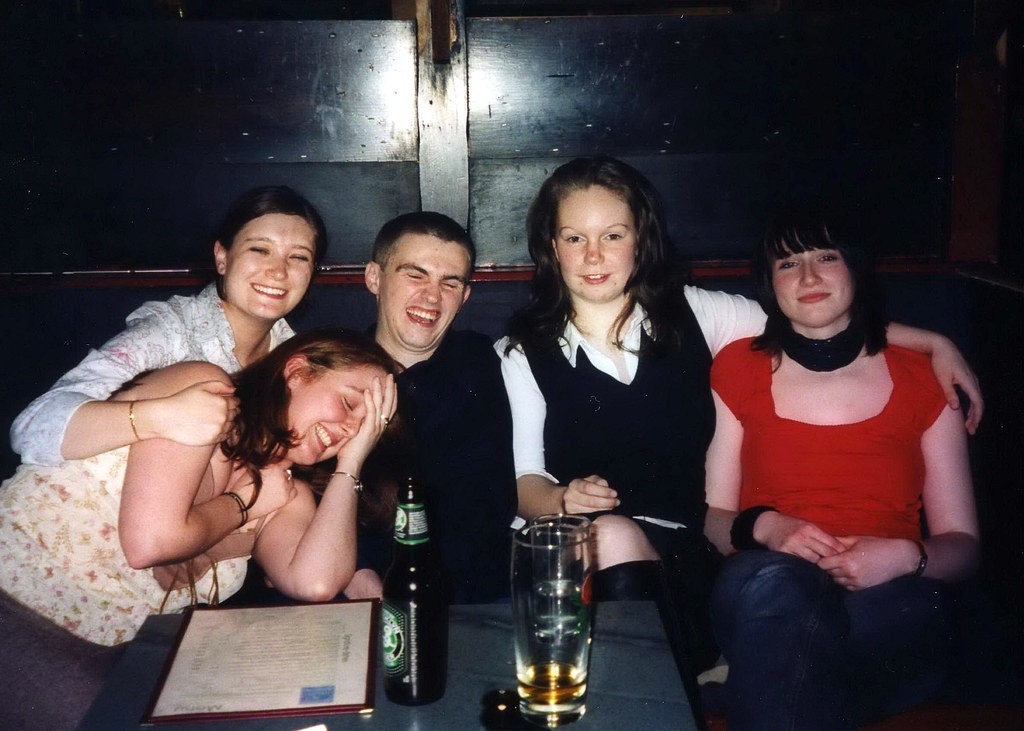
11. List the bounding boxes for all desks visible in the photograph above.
[75,591,699,731]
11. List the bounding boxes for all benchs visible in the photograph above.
[0,254,1023,731]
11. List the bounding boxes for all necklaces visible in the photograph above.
[778,321,865,371]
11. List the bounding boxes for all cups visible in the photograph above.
[510,524,591,727]
[519,515,592,652]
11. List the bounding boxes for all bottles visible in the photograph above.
[381,472,449,705]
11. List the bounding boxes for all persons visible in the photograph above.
[310,211,522,607]
[707,189,987,730]
[7,186,327,590]
[497,156,984,726]
[0,327,398,731]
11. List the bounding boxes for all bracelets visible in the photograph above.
[913,540,928,576]
[130,400,143,441]
[220,491,248,529]
[731,505,780,550]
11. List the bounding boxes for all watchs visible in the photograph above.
[328,472,363,500]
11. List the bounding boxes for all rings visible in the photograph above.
[381,415,389,425]
